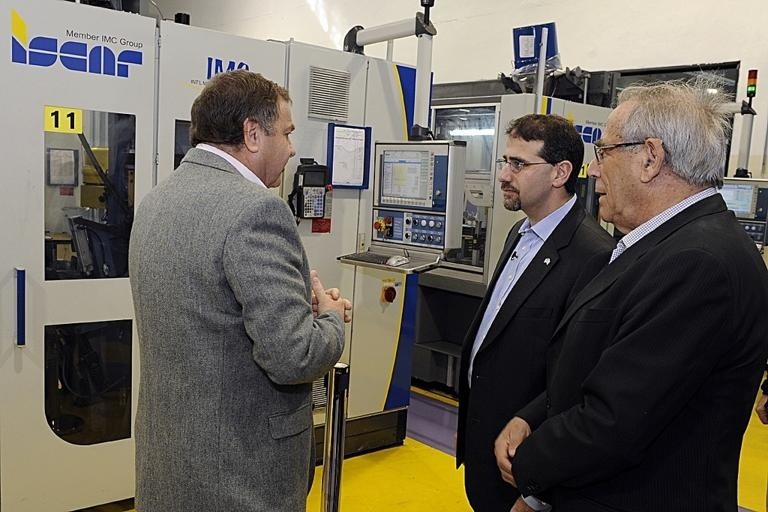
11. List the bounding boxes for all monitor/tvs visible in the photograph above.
[372,139,467,214]
[718,177,768,223]
[432,106,499,177]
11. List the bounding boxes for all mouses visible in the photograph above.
[386,254,409,268]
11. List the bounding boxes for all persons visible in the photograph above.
[490,81,768,512]
[453,111,621,511]
[126,69,355,510]
[755,373,768,425]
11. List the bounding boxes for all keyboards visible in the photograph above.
[336,251,390,266]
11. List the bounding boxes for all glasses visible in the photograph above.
[594,138,670,162]
[495,159,559,174]
